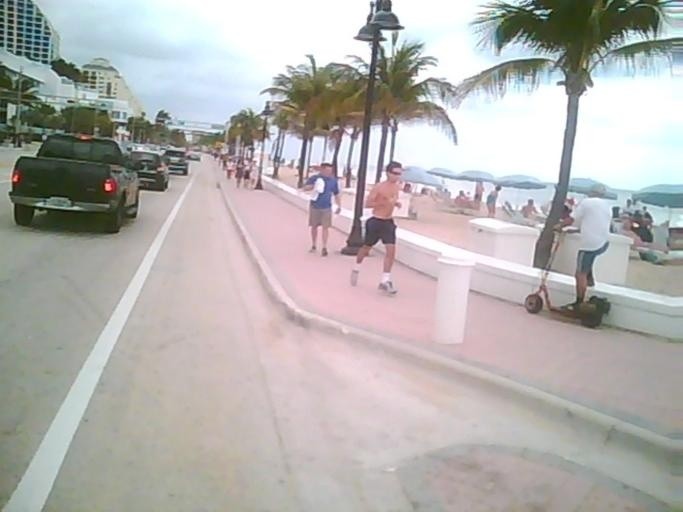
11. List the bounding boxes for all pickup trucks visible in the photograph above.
[8,132,140,235]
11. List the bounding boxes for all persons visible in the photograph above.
[220,153,258,188]
[552,183,612,303]
[403,181,654,246]
[303,163,342,256]
[350,160,402,294]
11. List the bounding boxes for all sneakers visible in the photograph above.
[587,279,596,286]
[322,248,328,255]
[562,304,582,308]
[308,246,320,253]
[378,281,398,294]
[351,269,358,287]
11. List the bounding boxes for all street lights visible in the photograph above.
[247,100,277,192]
[342,1,404,256]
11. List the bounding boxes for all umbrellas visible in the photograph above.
[632,184,683,208]
[554,178,617,200]
[426,168,546,189]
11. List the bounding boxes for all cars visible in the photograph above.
[116,141,205,191]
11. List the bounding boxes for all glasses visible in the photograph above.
[391,172,402,175]
